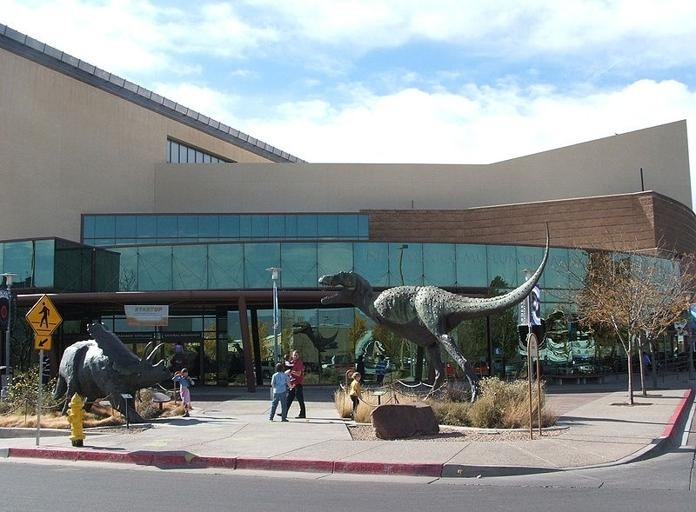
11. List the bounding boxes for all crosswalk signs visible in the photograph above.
[25,295,63,336]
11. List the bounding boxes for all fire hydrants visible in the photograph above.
[65,392,87,447]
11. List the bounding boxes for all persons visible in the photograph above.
[37,350,50,386]
[357,357,366,385]
[375,354,388,386]
[267,362,293,422]
[283,353,296,381]
[643,351,653,376]
[172,367,195,417]
[173,339,185,364]
[277,349,306,419]
[349,372,363,420]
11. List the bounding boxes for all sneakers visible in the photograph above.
[183,413,189,417]
[281,418,288,422]
[277,413,282,415]
[295,415,305,418]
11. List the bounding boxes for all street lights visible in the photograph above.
[265,267,282,364]
[0,273,17,374]
